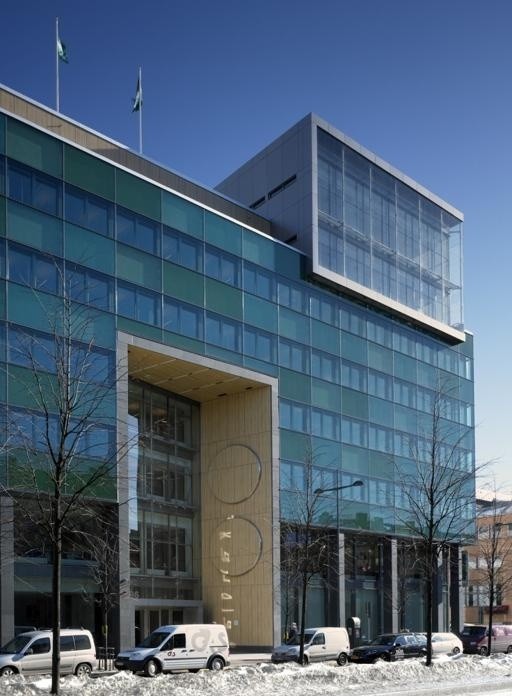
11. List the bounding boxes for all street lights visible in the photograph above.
[495,522,512,526]
[313,470,363,627]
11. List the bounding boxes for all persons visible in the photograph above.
[288,621,297,641]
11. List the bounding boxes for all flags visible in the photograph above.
[131,80,142,113]
[57,36,69,63]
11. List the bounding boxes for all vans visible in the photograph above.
[0,627,97,678]
[115,624,231,677]
[271,627,350,666]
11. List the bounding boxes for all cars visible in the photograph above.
[348,623,512,664]
